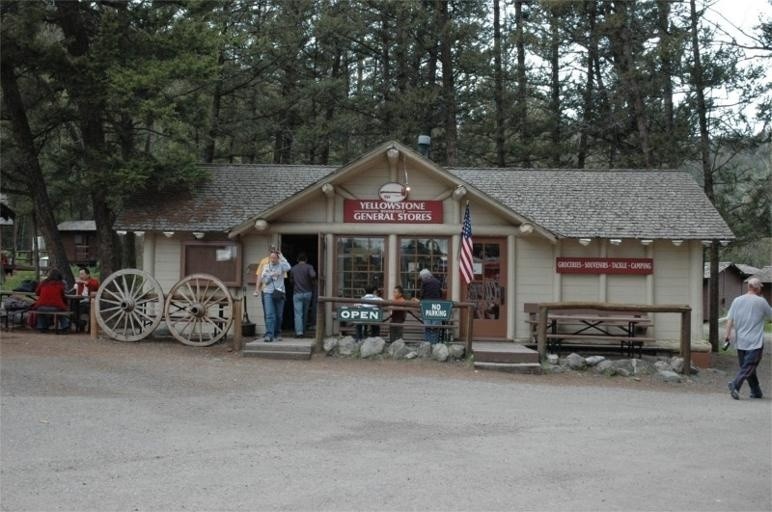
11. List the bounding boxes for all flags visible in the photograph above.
[458,202,474,285]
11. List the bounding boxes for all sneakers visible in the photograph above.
[727,381,740,400]
[40,320,87,333]
[263,334,306,341]
[750,393,763,399]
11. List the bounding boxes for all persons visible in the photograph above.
[260,250,292,341]
[32,267,72,334]
[724,278,772,401]
[0,252,10,276]
[289,253,317,338]
[410,268,444,344]
[252,244,288,339]
[64,267,100,334]
[389,284,407,344]
[356,283,381,337]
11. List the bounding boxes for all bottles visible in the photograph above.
[74,283,78,295]
[721,341,731,352]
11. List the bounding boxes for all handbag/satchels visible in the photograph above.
[272,289,285,301]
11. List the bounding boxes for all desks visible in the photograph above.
[546,314,650,356]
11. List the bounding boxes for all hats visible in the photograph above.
[747,278,764,288]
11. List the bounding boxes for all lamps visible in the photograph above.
[578,237,730,249]
[116,230,206,242]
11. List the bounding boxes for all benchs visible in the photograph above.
[524,320,654,358]
[537,333,655,358]
[4,290,96,333]
[349,322,457,342]
